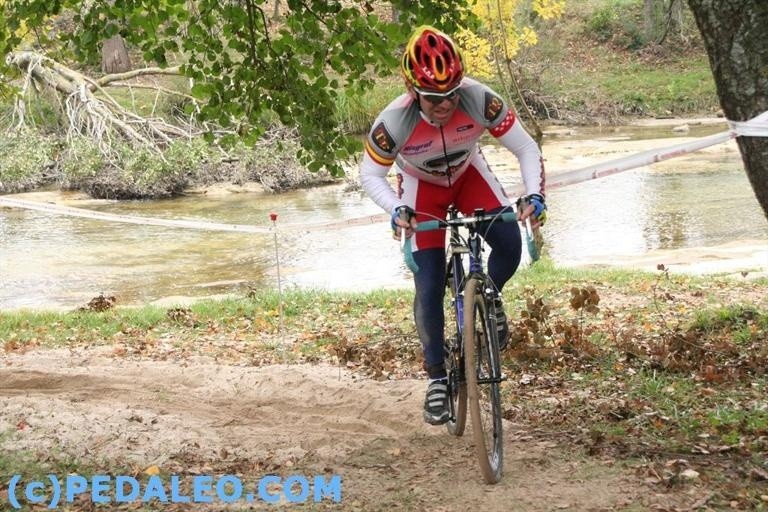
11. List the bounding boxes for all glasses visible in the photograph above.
[412,84,463,105]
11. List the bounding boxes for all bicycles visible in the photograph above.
[400,195,541,484]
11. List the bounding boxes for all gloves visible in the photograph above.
[390,204,416,242]
[526,194,549,227]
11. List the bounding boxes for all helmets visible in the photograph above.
[402,24,465,92]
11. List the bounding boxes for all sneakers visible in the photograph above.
[488,293,509,351]
[421,378,452,426]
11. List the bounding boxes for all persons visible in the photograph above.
[359,24,547,426]
